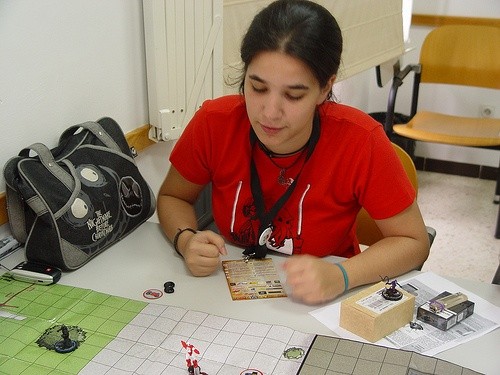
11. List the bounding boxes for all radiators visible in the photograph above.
[141,0,220,141]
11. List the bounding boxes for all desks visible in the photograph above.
[0,221,500,375]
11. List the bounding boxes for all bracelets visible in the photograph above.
[335,263,348,290]
[173,228,196,257]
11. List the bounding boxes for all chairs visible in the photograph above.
[355,143,436,271]
[386,24,500,238]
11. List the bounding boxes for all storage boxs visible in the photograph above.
[0,234,22,259]
[417,291,474,331]
[340,282,415,343]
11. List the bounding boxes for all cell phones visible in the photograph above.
[10,260,62,285]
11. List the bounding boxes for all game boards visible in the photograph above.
[0,272,489,375]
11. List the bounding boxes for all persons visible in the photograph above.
[155,0,429,306]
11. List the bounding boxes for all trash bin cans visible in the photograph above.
[367,112,411,152]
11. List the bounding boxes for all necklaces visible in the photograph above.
[261,145,306,184]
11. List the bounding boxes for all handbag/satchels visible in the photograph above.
[2,117,158,273]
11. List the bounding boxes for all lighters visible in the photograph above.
[429,292,468,312]
